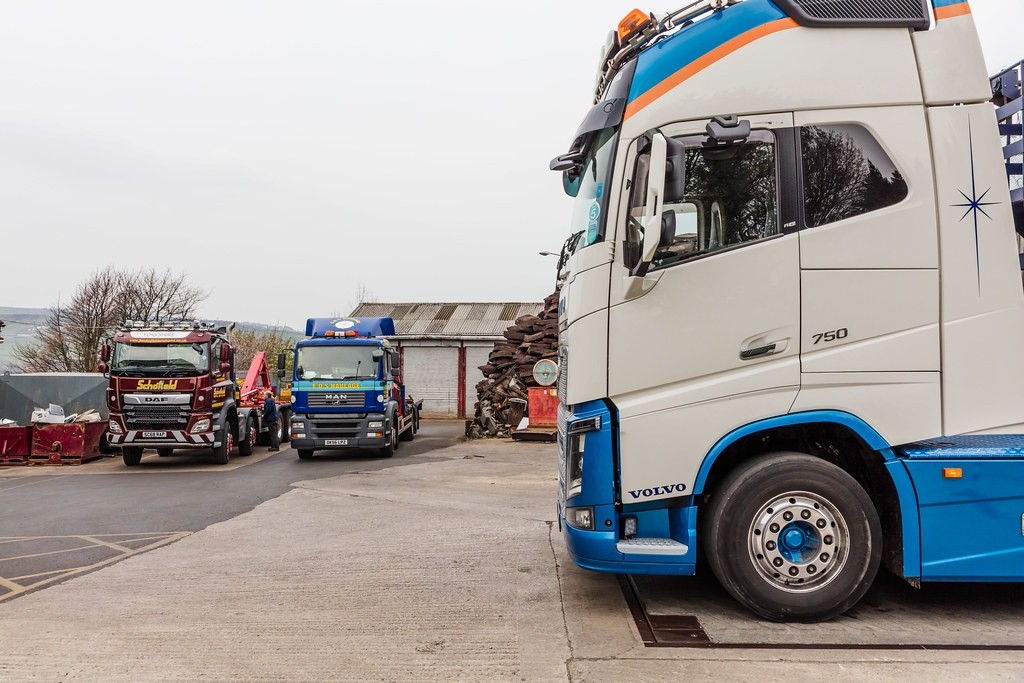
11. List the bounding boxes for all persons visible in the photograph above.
[262,392,279,451]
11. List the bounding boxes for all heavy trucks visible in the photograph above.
[554,0,1024,624]
[277,317,424,459]
[98,321,293,465]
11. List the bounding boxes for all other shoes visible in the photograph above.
[268,446,279,451]
